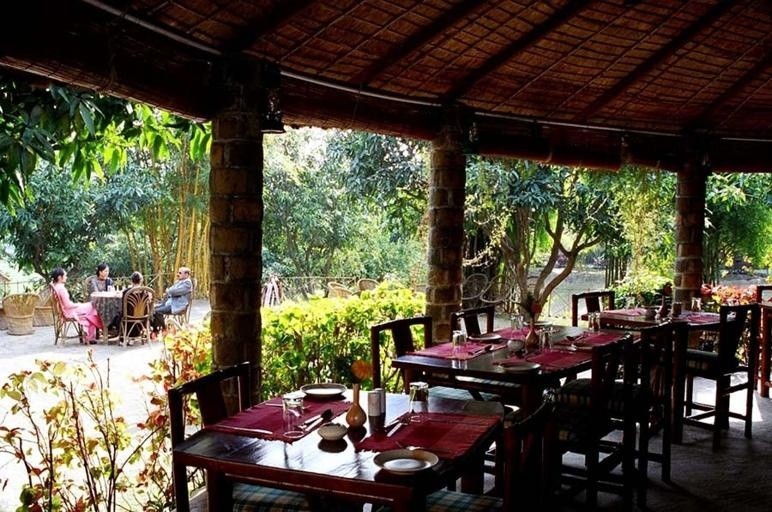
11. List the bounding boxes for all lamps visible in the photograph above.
[236,57,290,137]
[455,96,481,147]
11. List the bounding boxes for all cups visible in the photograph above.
[450,329,470,359]
[510,314,524,334]
[624,296,635,315]
[409,382,429,415]
[689,298,701,318]
[283,400,306,440]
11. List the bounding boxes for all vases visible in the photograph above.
[659,297,671,317]
[645,309,657,319]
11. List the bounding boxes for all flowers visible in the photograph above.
[518,294,548,317]
[334,355,373,384]
[641,290,659,309]
[659,283,674,296]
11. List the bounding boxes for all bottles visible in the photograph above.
[672,301,684,315]
[539,327,554,346]
[368,388,385,418]
[588,312,601,332]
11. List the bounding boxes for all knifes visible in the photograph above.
[216,423,273,435]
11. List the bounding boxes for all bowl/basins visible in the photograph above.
[316,423,348,439]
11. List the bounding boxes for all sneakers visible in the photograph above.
[84,329,167,347]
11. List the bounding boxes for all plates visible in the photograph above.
[570,342,603,350]
[470,333,503,342]
[371,445,440,475]
[523,320,552,327]
[300,380,348,397]
[499,359,542,373]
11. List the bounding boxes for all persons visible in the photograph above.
[50,267,104,345]
[117,270,149,347]
[87,263,114,302]
[148,267,193,335]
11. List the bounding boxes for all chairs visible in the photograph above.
[168,360,578,512]
[673,304,760,440]
[371,305,678,507]
[0,275,160,346]
[753,285,772,391]
[572,290,616,328]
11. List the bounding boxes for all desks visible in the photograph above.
[761,305,772,397]
[581,306,752,415]
